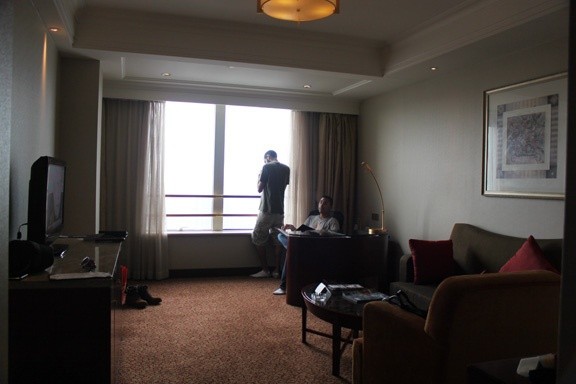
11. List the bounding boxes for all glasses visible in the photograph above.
[81,255,90,272]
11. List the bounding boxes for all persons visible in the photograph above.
[273,196,340,296]
[249,150,290,279]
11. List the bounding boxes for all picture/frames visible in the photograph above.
[481,71,568,200]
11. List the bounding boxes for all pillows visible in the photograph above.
[499,235,557,273]
[409,238,453,285]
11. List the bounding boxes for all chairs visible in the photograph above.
[308,211,344,233]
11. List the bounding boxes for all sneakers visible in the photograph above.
[271,272,279,278]
[248,270,269,278]
[272,288,285,294]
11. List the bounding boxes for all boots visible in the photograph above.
[124,286,147,308]
[138,285,161,304]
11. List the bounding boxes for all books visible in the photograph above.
[328,283,363,291]
[341,288,388,305]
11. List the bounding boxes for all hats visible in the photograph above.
[9,239,53,276]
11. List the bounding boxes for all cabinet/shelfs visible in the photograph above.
[9,235,122,384]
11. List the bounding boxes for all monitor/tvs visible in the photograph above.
[27,156,69,257]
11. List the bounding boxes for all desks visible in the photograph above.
[271,226,352,304]
[467,350,557,383]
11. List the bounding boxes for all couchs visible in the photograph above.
[389,223,564,317]
[350,270,562,383]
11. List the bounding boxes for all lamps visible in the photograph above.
[360,160,388,236]
[256,0,340,21]
